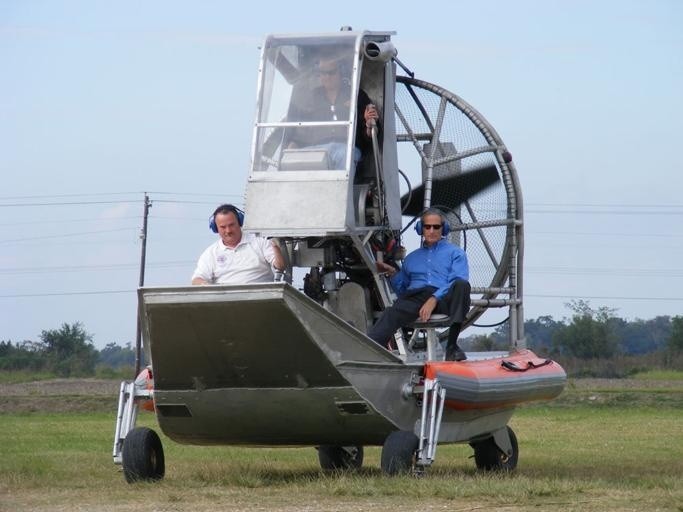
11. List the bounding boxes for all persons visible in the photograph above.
[192,204,290,285]
[366,207,471,361]
[287,54,379,182]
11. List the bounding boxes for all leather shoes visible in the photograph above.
[445,343,467,361]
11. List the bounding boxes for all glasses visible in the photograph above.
[424,224,443,230]
[330,104,338,121]
[320,69,337,76]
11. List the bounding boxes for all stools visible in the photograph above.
[407,313,449,365]
